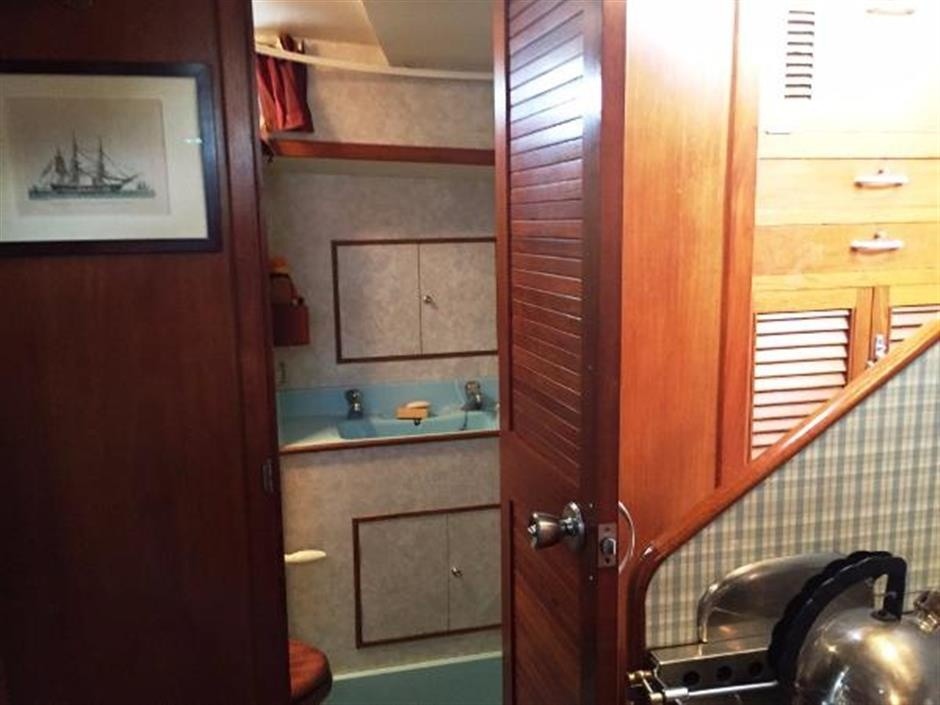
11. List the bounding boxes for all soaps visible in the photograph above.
[408,399,433,411]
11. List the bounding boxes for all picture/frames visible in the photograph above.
[1,56,225,260]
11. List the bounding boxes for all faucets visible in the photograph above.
[461,380,488,411]
[344,389,366,419]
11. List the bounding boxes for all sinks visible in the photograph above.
[337,409,504,444]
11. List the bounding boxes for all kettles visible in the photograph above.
[769,550,940,704]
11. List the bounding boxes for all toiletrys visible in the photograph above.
[269,255,298,309]
